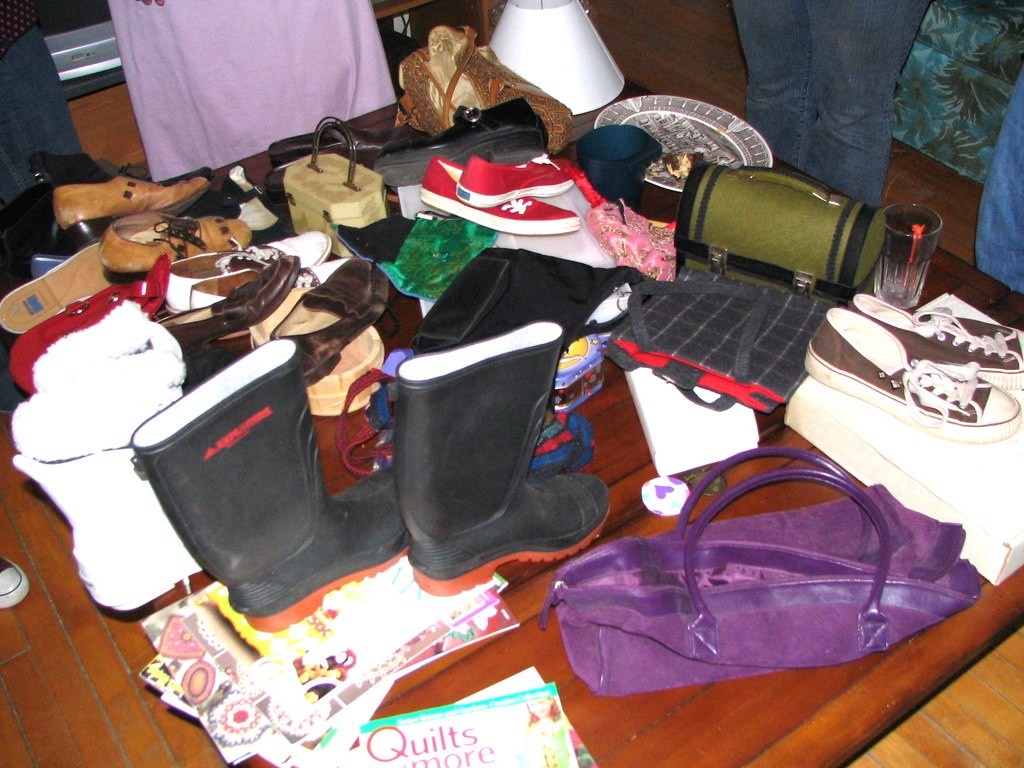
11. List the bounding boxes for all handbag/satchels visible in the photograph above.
[603,265,838,415]
[284,115,387,258]
[539,445,984,698]
[674,162,885,305]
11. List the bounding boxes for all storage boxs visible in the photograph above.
[555,334,606,413]
[784,292,1023,587]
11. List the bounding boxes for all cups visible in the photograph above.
[874,203,944,313]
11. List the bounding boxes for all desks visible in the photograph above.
[152,78,1024,768]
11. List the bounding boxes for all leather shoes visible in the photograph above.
[0,172,390,389]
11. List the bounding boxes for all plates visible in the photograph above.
[592,93,774,198]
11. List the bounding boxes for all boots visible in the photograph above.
[393,319,611,596]
[12,298,214,618]
[132,338,415,632]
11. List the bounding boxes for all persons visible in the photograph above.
[730,0,931,209]
[0,0,398,206]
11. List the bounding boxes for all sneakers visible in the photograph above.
[849,294,1024,391]
[803,308,1022,445]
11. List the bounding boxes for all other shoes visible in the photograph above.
[0,556,29,610]
[420,157,581,237]
[221,164,282,246]
[455,155,576,210]
[373,95,549,190]
[401,23,573,154]
